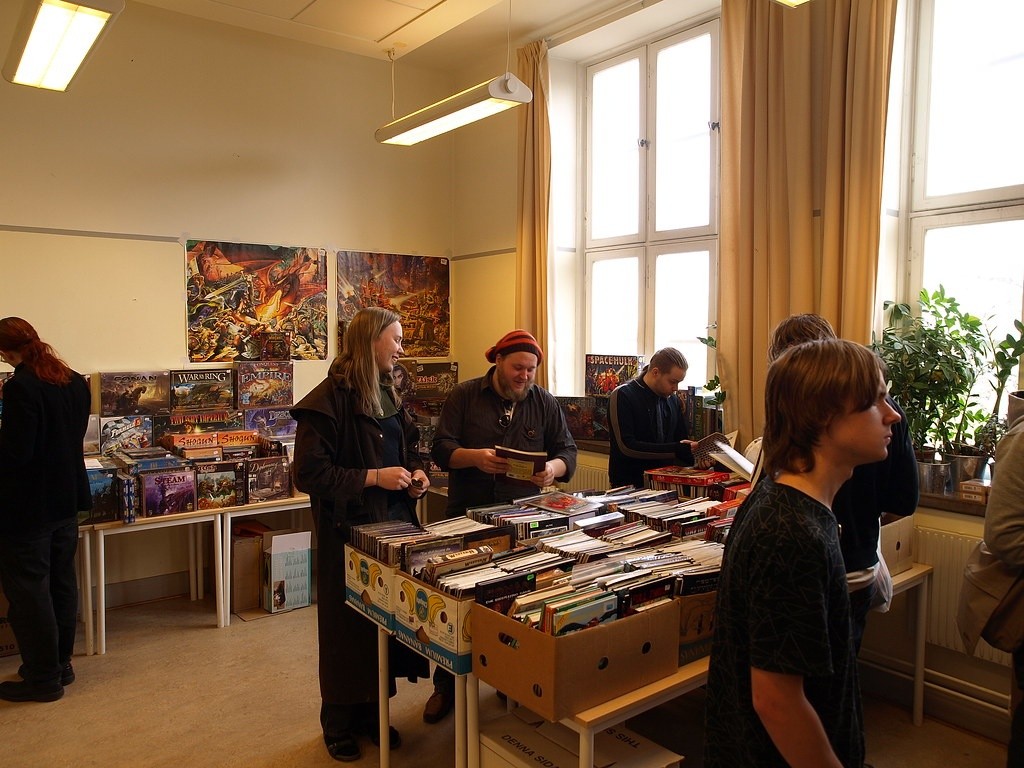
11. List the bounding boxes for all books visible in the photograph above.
[708,436,763,481]
[494,444,547,503]
[350,465,754,636]
[673,386,721,440]
[689,429,738,465]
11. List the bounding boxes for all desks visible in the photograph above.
[77,491,312,657]
[466,562,934,768]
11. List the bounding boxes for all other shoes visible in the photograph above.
[423,691,454,724]
[495,690,519,708]
[19,659,74,686]
[351,714,401,748]
[0,673,64,702]
[321,719,361,762]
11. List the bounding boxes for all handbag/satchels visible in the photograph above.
[956,541,1024,658]
[870,516,893,613]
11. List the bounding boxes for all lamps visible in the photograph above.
[0,0,126,93]
[374,0,533,147]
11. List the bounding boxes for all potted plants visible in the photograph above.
[866,284,1024,496]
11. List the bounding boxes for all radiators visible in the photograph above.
[551,464,610,494]
[904,526,1012,668]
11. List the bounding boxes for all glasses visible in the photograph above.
[410,478,424,491]
[498,399,514,428]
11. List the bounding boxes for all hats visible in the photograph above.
[485,329,543,366]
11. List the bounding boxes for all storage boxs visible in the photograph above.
[480,705,685,768]
[550,354,723,442]
[262,529,312,614]
[205,521,263,613]
[960,491,988,506]
[75,331,309,527]
[959,478,992,495]
[343,542,715,724]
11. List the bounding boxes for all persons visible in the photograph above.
[0,315,92,701]
[425,329,575,726]
[700,339,901,768]
[289,307,431,762]
[607,348,714,491]
[983,390,1024,768]
[750,314,919,655]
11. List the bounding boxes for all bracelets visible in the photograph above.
[375,466,380,487]
